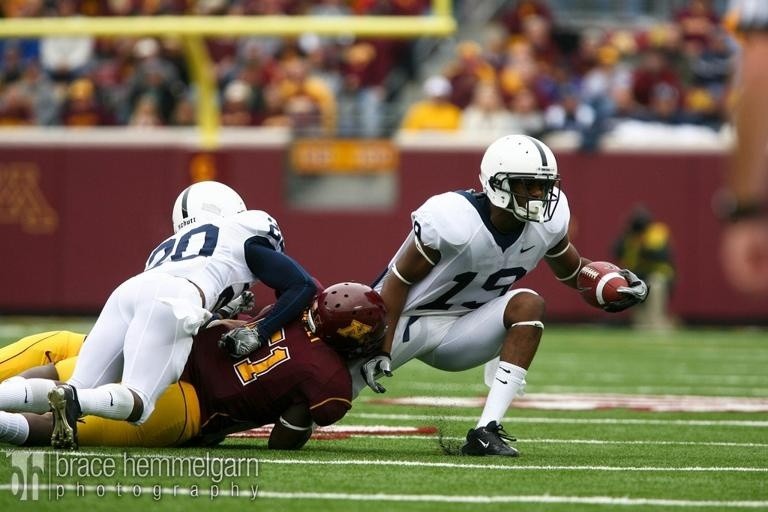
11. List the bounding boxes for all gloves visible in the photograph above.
[216,290,255,318]
[360,351,393,393]
[603,268,649,314]
[217,325,262,357]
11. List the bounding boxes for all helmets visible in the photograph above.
[478,133,556,223]
[171,180,248,234]
[306,281,389,359]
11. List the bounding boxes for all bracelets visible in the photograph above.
[719,192,768,226]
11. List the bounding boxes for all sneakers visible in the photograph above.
[47,384,83,451]
[462,420,520,456]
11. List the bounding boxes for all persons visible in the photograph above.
[0,179,318,429]
[713,0,768,297]
[0,309,256,383]
[0,281,392,450]
[342,132,649,458]
[617,209,681,331]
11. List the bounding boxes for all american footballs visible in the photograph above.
[577,262,629,309]
